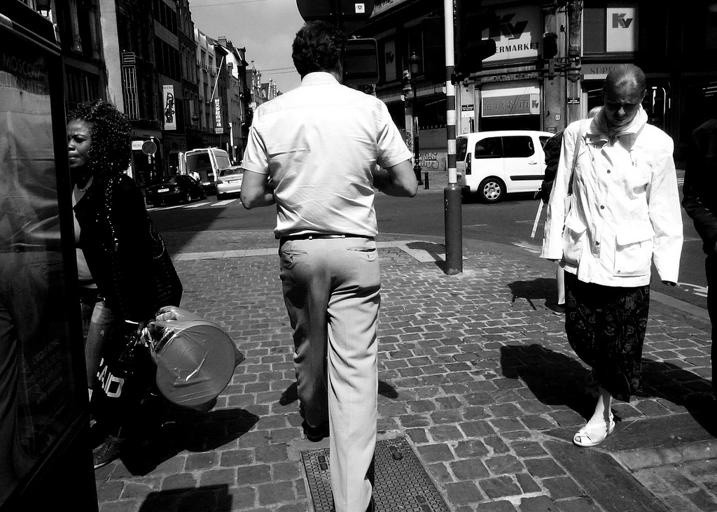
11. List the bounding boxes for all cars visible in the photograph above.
[215,166,246,202]
[144,174,208,205]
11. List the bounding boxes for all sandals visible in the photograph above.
[573,413,615,448]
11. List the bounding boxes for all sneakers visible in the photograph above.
[93,435,125,469]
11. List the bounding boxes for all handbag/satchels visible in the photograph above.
[540,129,574,204]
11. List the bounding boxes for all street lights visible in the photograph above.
[406,51,425,187]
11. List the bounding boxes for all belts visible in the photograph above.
[289,233,355,240]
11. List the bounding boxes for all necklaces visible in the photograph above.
[74,181,90,193]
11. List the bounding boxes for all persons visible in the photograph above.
[538,104,605,311]
[0,32,70,510]
[241,22,420,511]
[685,119,716,405]
[540,64,683,446]
[66,98,183,470]
[189,168,200,183]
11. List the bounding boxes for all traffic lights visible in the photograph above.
[545,33,558,58]
[457,0,496,72]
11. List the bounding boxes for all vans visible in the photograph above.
[456,126,559,207]
[177,147,232,187]
